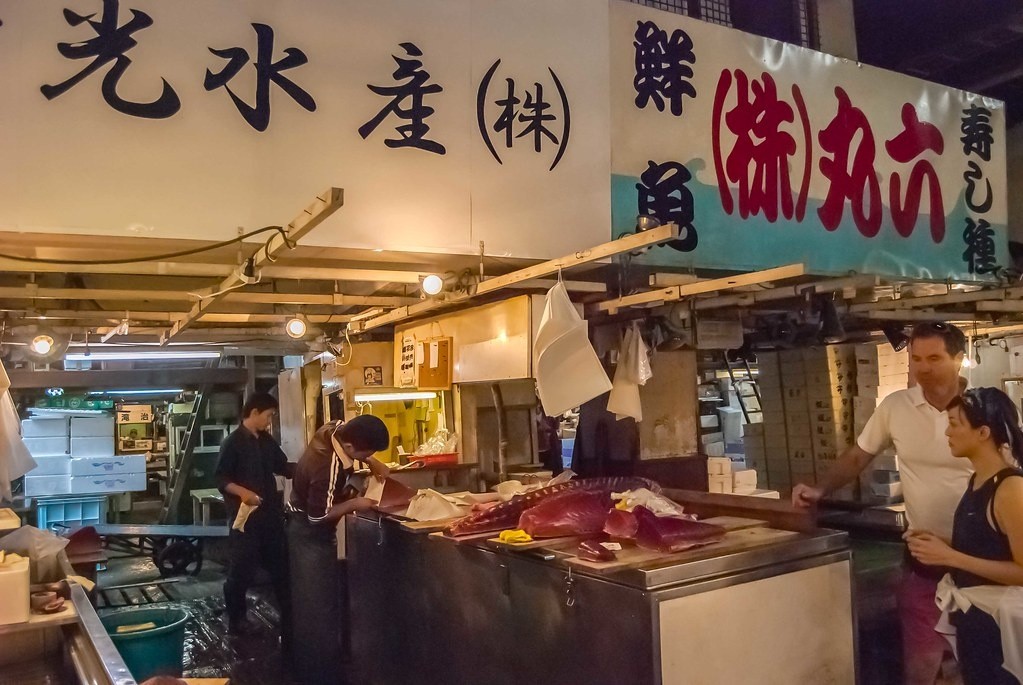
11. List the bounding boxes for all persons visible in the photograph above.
[902,387,1023,685]
[284,414,391,685]
[214,391,299,636]
[792,320,1023,685]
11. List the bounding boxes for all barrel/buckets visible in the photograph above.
[99,607,190,685]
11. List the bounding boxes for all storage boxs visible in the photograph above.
[20,408,147,571]
[697,346,910,507]
[1,557,30,625]
[30,553,57,583]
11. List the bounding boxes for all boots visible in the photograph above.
[224,582,264,635]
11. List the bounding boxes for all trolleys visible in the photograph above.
[50,522,202,578]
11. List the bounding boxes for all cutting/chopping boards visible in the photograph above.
[400,515,799,574]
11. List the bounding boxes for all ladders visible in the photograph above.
[725,354,761,424]
[160,359,218,526]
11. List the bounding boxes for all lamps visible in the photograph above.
[636,215,661,233]
[815,299,848,344]
[349,392,439,402]
[994,265,1023,283]
[880,325,909,352]
[32,326,57,354]
[287,319,322,339]
[63,350,221,361]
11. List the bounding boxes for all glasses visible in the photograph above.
[916,321,960,350]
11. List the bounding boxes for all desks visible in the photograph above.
[190,489,224,527]
